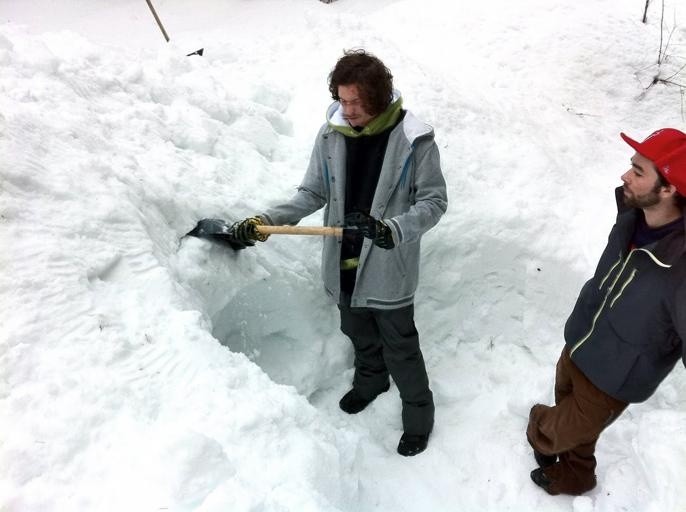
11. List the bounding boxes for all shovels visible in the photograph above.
[188,217,368,250]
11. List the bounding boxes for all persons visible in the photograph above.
[227,48,448,456]
[526,128,686,495]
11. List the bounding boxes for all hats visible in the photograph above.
[619,128,686,198]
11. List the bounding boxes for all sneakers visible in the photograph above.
[529,449,559,492]
[397,430,428,457]
[339,380,390,414]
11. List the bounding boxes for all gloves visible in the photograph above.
[226,214,270,248]
[348,211,395,250]
